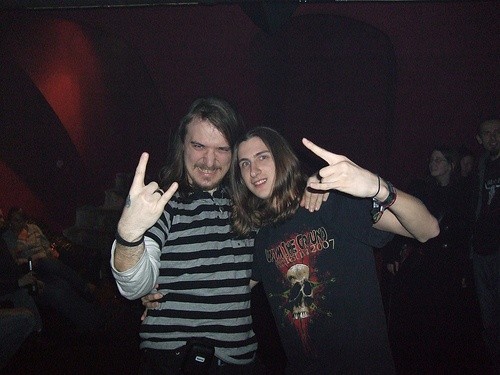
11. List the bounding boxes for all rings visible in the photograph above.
[154,188,163,197]
[314,168,324,181]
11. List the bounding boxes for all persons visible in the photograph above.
[141,126,442,375]
[386,116,500,375]
[0,206,108,340]
[109,98,330,375]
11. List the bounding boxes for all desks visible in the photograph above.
[62,227,123,306]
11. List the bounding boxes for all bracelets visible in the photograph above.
[113,230,144,246]
[371,172,398,224]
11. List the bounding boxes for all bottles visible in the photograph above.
[27,256,37,296]
